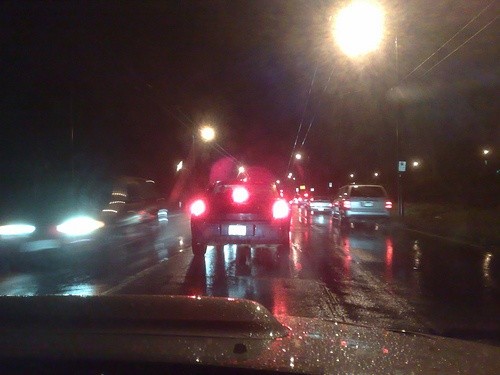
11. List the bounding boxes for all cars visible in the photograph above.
[289,193,315,208]
[307,195,332,215]
[0,176,169,274]
[190,180,292,256]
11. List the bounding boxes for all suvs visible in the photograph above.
[331,184,393,228]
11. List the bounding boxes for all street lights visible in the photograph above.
[335,3,404,219]
